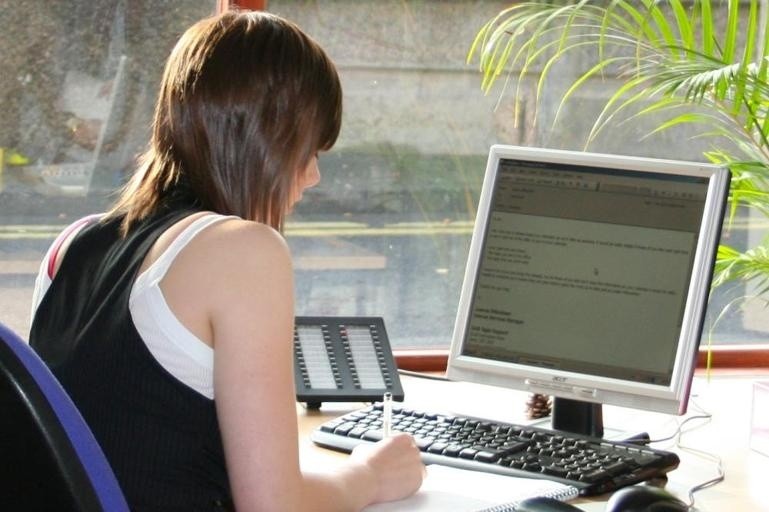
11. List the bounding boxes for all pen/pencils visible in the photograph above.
[383,392,393,440]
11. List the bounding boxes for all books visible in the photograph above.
[356,460,580,512]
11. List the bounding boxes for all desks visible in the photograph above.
[295,368,769,512]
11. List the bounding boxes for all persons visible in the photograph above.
[28,7,425,512]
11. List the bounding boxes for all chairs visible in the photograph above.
[0,316,132,512]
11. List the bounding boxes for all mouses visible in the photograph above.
[608,482,691,512]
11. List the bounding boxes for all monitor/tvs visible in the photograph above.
[445,144,733,444]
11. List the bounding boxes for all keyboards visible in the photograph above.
[312,401,679,495]
